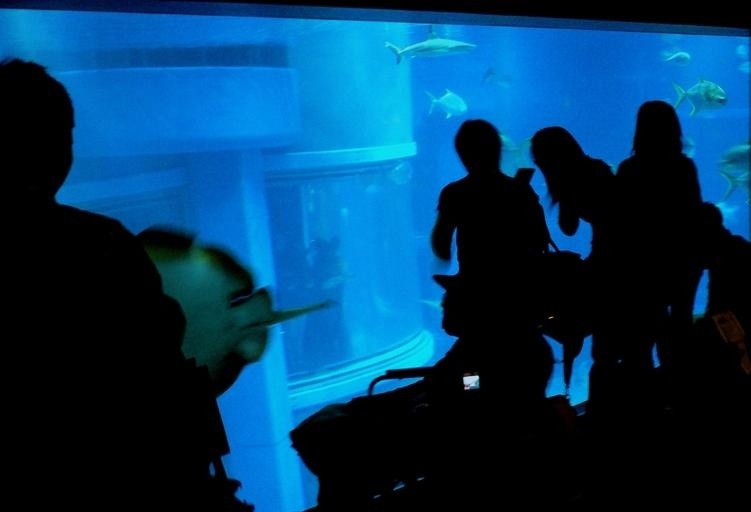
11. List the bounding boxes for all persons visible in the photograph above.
[293,98,750,511]
[0,56,254,510]
